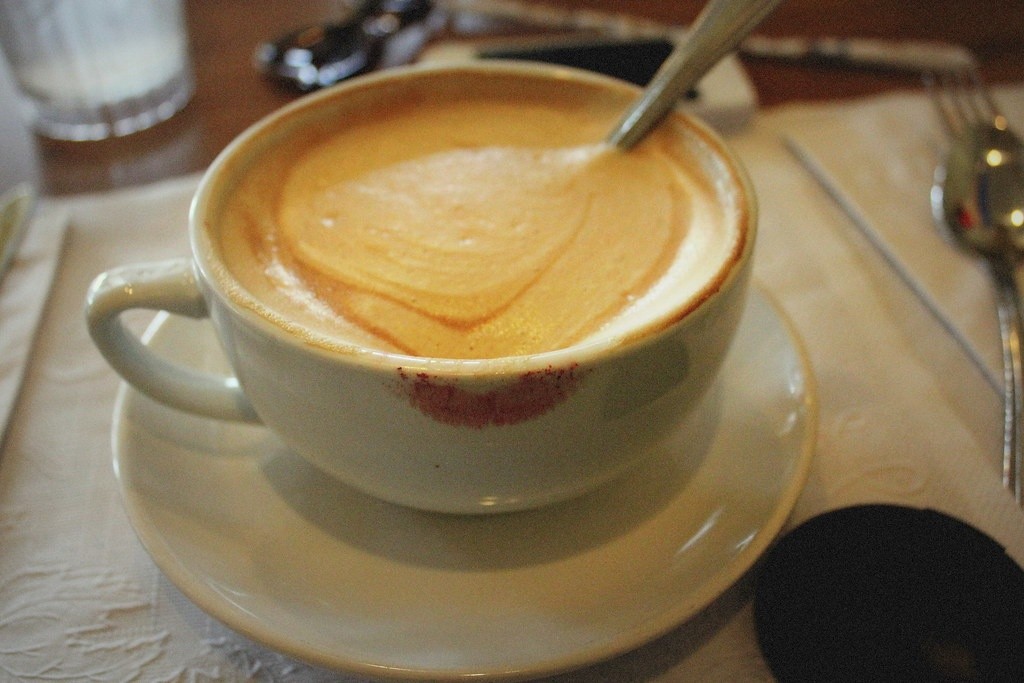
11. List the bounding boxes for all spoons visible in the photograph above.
[930,125,1024,507]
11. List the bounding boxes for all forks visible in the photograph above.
[917,47,1024,160]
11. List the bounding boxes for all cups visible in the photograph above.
[0,1,198,140]
[84,60,757,524]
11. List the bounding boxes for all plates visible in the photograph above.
[110,276,821,679]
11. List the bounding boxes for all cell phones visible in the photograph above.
[420,26,759,132]
